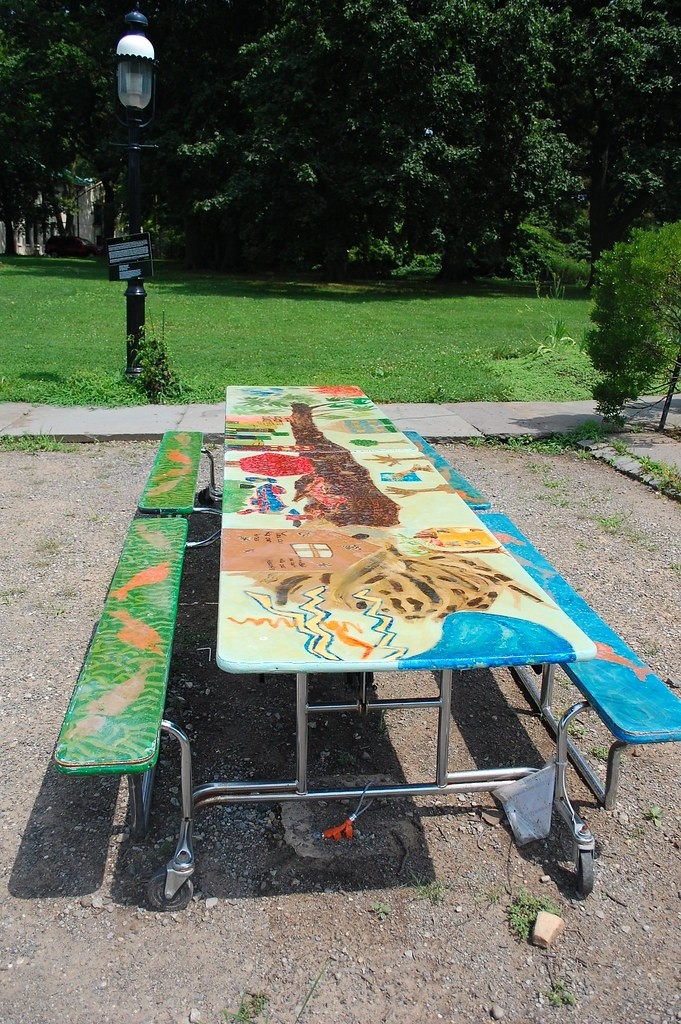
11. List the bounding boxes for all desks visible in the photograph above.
[148,386,598,913]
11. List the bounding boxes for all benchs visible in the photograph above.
[475,513,681,744]
[401,430,491,511]
[137,430,203,515]
[51,517,189,779]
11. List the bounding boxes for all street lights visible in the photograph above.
[104,11,161,381]
